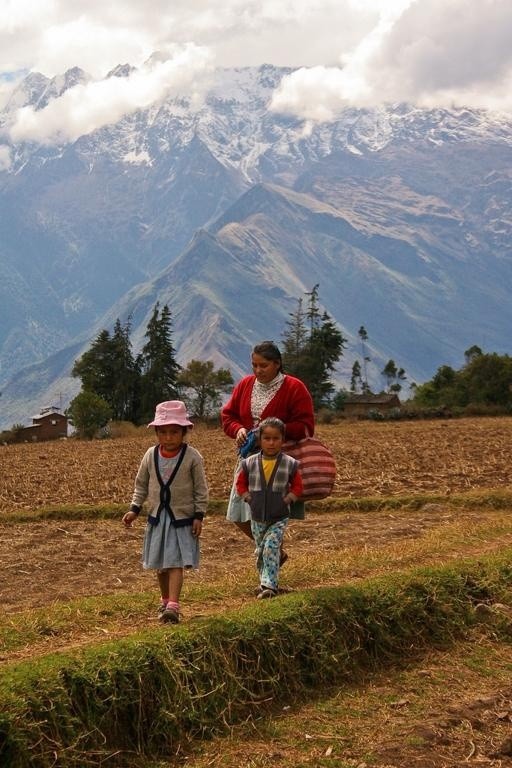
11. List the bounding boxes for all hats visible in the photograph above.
[147,401,194,432]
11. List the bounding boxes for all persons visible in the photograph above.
[234,415,303,601]
[220,340,314,568]
[122,399,208,625]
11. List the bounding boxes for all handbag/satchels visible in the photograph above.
[280,429,336,503]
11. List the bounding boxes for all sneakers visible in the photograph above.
[280,549,288,567]
[263,589,277,599]
[162,608,179,624]
